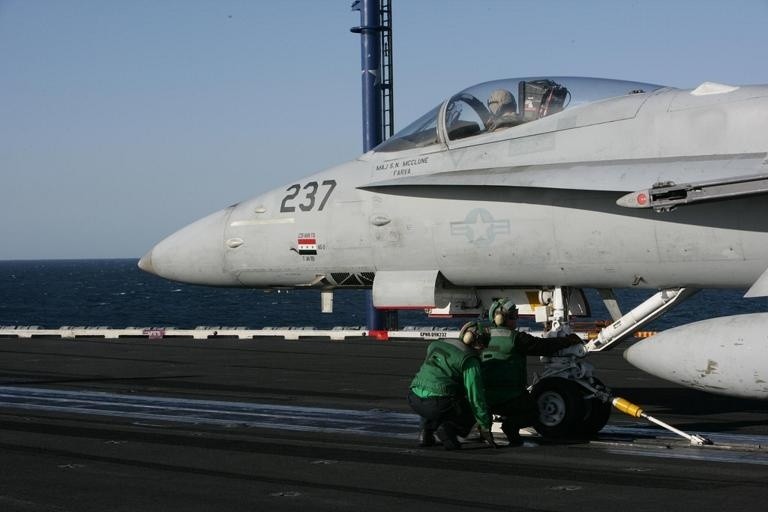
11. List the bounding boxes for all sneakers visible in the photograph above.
[501,424,524,444]
[433,427,456,450]
[419,430,432,447]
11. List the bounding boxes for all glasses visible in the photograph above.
[506,308,519,320]
[479,335,491,347]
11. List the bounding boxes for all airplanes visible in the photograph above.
[134,75,768,439]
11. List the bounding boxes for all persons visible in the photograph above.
[406,321,504,453]
[476,297,586,447]
[482,88,519,132]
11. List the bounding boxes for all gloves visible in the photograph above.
[481,431,498,448]
[566,332,584,347]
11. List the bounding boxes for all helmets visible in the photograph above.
[458,320,492,348]
[488,298,515,325]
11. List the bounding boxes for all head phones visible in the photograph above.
[494,301,515,325]
[463,325,479,345]
